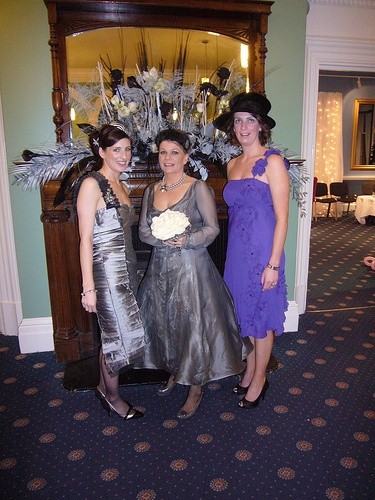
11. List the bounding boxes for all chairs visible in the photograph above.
[331,182,356,216]
[317,182,338,223]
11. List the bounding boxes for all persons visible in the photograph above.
[76,124,147,419]
[215,92,290,407]
[138,129,254,420]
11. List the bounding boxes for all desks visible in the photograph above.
[354,193,375,225]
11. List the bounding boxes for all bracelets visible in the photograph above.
[81,289,98,296]
[267,264,280,271]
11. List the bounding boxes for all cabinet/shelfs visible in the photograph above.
[33,170,234,364]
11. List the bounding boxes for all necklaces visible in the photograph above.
[160,173,186,192]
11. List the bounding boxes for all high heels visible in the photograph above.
[176,389,205,420]
[96,387,133,409]
[237,376,269,410]
[155,380,179,396]
[232,379,249,395]
[101,395,144,420]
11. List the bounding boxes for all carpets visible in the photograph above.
[63,358,171,389]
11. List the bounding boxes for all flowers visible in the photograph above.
[7,9,309,209]
[149,209,194,257]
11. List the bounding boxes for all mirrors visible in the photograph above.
[350,98,375,170]
[41,0,272,159]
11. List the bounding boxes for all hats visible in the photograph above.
[212,93,276,134]
